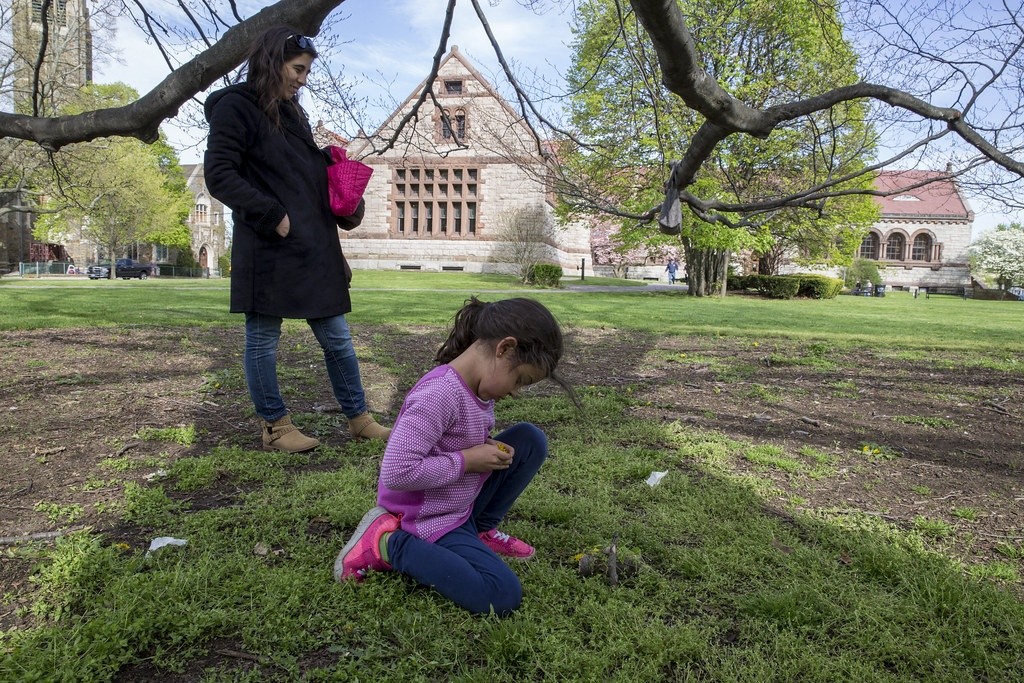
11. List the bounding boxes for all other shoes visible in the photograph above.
[348,410,392,441]
[260,415,321,454]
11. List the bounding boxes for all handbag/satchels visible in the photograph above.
[326,145,374,217]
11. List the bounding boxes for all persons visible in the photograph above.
[204,25,394,453]
[334,297,563,619]
[665,259,678,285]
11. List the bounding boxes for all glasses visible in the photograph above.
[287,34,315,48]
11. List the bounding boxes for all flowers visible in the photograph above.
[495,442,509,463]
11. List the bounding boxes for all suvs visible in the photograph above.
[86,258,154,282]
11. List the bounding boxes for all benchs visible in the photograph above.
[924,287,967,300]
[854,291,873,296]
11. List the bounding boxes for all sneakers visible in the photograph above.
[334,506,403,583]
[477,527,536,559]
